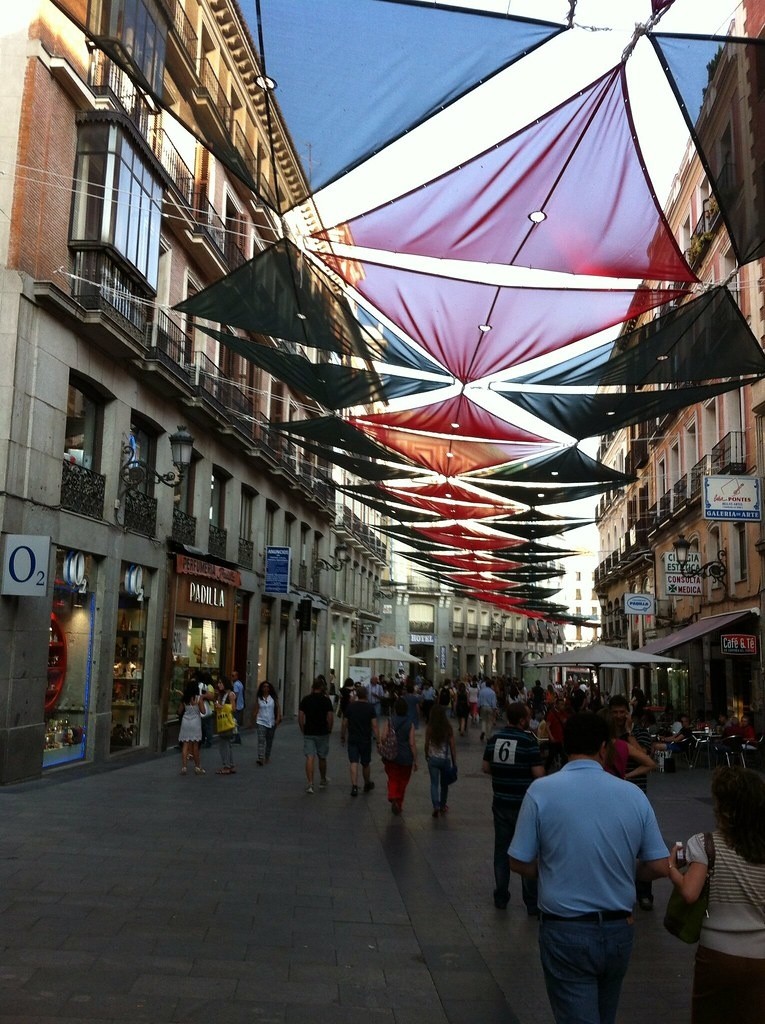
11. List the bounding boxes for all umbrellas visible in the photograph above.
[533,642,683,711]
[346,646,424,674]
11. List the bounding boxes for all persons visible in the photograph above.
[180,671,765,1024]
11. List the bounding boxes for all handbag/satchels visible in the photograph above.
[232,718,240,734]
[200,683,213,718]
[662,831,716,944]
[376,717,399,760]
[216,704,235,732]
[441,766,457,786]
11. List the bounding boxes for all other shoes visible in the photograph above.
[257,759,263,764]
[318,781,329,789]
[391,799,403,815]
[305,784,315,794]
[341,737,345,742]
[351,785,358,796]
[231,738,241,744]
[638,896,653,910]
[480,732,485,741]
[494,888,507,909]
[432,805,448,819]
[527,906,540,915]
[364,782,374,792]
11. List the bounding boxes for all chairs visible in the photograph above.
[655,723,765,770]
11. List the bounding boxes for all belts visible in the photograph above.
[540,911,632,923]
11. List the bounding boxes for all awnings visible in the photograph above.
[634,606,759,655]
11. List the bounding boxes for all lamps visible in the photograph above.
[311,540,351,578]
[373,579,396,600]
[113,424,194,512]
[672,533,727,590]
[597,590,626,620]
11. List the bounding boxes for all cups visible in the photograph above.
[705,727,709,732]
[716,726,722,735]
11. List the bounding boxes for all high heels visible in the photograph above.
[195,767,206,775]
[179,767,187,775]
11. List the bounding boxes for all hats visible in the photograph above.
[312,678,327,690]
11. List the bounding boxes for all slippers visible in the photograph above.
[215,768,237,775]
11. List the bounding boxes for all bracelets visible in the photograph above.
[668,865,676,870]
